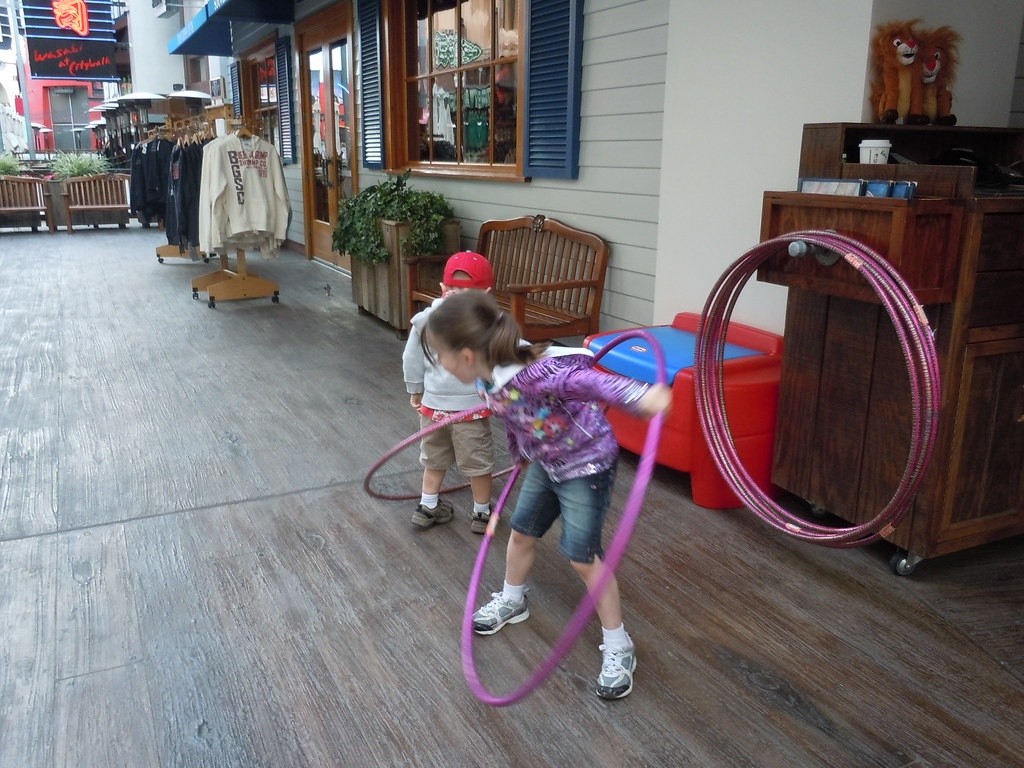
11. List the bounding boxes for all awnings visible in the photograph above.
[168,0,295,56]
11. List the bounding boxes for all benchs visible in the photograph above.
[0,176,56,233]
[401,214,610,343]
[58,171,164,235]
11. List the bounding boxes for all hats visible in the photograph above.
[443,250,494,289]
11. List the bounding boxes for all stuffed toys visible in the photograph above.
[868,18,964,125]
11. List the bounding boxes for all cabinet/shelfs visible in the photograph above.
[756,121,1024,576]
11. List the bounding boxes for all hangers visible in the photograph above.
[141,115,253,149]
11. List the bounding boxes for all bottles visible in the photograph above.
[842,154,848,163]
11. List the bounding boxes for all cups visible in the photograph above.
[859,140,892,164]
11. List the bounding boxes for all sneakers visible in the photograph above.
[411,499,453,528]
[470,504,496,533]
[595,632,637,700]
[472,592,530,635]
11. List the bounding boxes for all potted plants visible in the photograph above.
[47,149,130,230]
[1,151,42,234]
[328,168,461,340]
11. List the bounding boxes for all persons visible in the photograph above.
[403,249,533,534]
[420,291,673,700]
